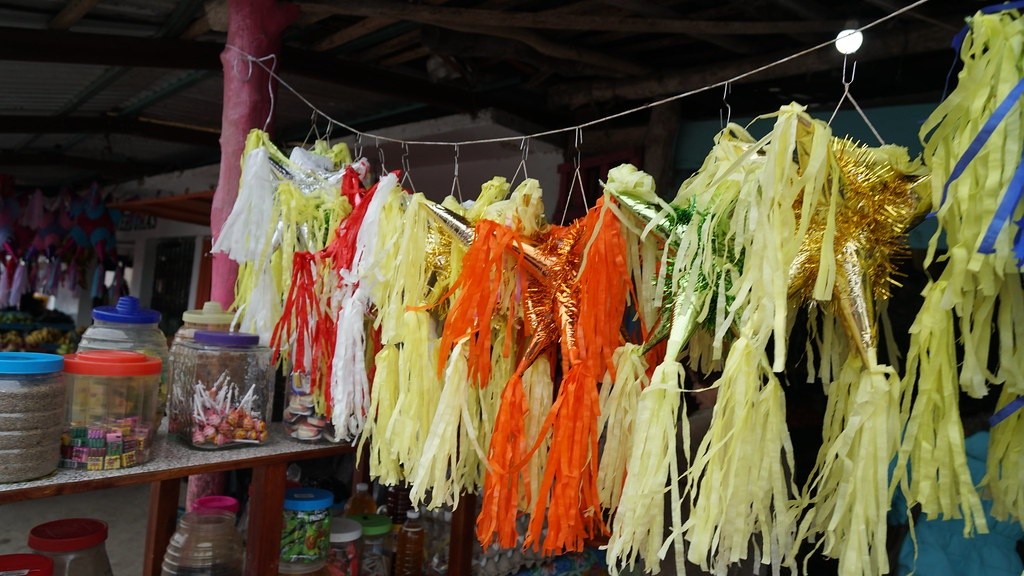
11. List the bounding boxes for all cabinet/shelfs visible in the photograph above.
[0,411,487,575]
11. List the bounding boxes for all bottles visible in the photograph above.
[395,509,423,576]
[325,516,361,576]
[384,465,413,539]
[170,330,274,451]
[343,481,377,516]
[280,487,334,564]
[0,350,67,483]
[59,350,165,472]
[168,302,241,417]
[26,516,113,575]
[352,511,393,576]
[76,294,171,436]
[160,509,242,576]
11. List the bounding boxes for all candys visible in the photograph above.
[170,371,267,446]
[280,511,331,564]
[62,417,152,470]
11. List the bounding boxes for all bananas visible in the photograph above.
[0,328,77,352]
